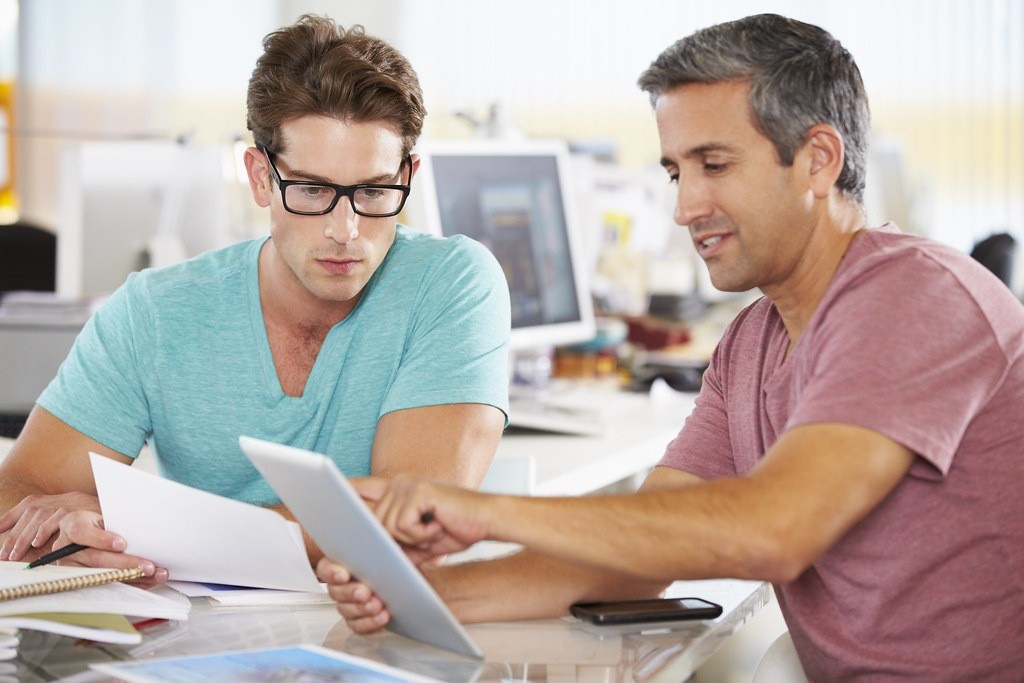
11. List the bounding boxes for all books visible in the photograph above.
[0,561,191,683]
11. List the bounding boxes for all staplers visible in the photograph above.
[617,355,710,395]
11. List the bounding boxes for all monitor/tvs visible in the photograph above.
[411,137,596,394]
[79,180,231,306]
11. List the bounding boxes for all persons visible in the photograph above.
[317,14,1024,683]
[0,14,512,590]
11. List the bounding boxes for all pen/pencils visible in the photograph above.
[21,543,92,570]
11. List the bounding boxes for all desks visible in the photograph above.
[124,382,810,683]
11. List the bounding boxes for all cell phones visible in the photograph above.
[571,598,722,626]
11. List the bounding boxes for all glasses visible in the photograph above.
[262,145,412,217]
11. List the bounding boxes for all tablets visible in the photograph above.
[239,436,484,663]
